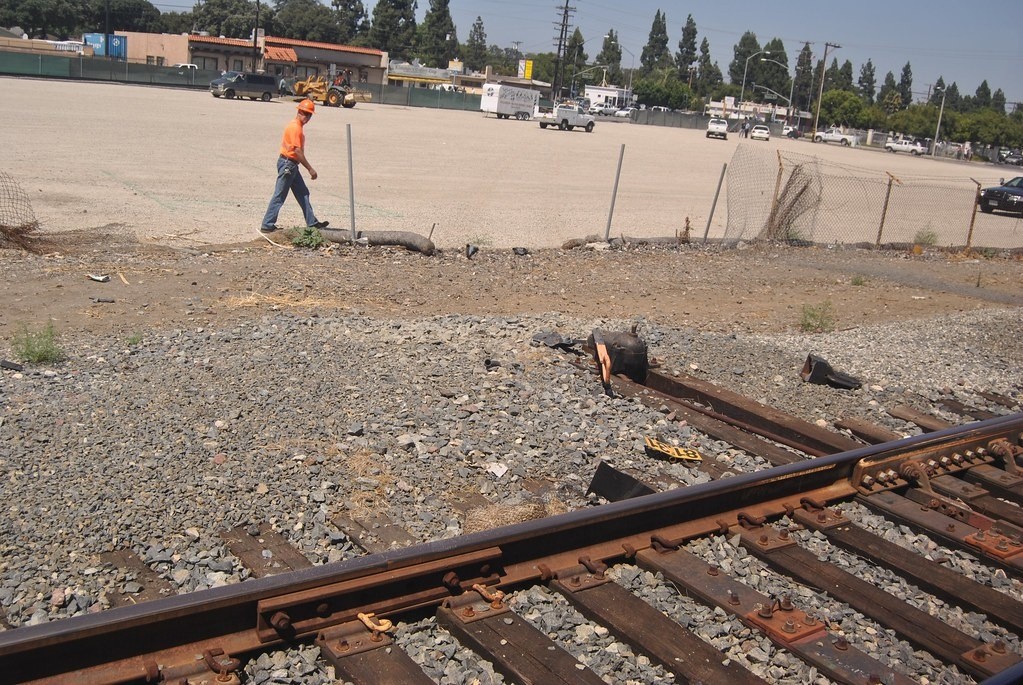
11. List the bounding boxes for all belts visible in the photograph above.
[279,154,299,165]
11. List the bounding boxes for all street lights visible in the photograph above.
[610,40,636,107]
[931,86,947,157]
[571,34,610,98]
[760,57,797,108]
[737,50,772,120]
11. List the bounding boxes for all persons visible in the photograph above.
[261,100,329,233]
[739,121,750,138]
[279,79,286,97]
[458,87,475,94]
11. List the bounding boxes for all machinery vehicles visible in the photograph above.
[293,68,372,109]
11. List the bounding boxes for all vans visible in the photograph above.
[209,70,279,102]
[652,105,670,113]
[172,63,198,70]
[615,107,638,117]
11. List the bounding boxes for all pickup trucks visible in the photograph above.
[814,128,858,148]
[705,119,729,140]
[534,103,595,133]
[886,139,929,156]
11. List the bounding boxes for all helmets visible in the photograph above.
[296,99,317,115]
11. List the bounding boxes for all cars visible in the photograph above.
[588,102,621,117]
[536,96,591,114]
[782,126,793,136]
[1004,154,1023,165]
[977,176,1023,218]
[751,124,771,141]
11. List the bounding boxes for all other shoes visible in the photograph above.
[260,225,284,233]
[309,221,330,228]
